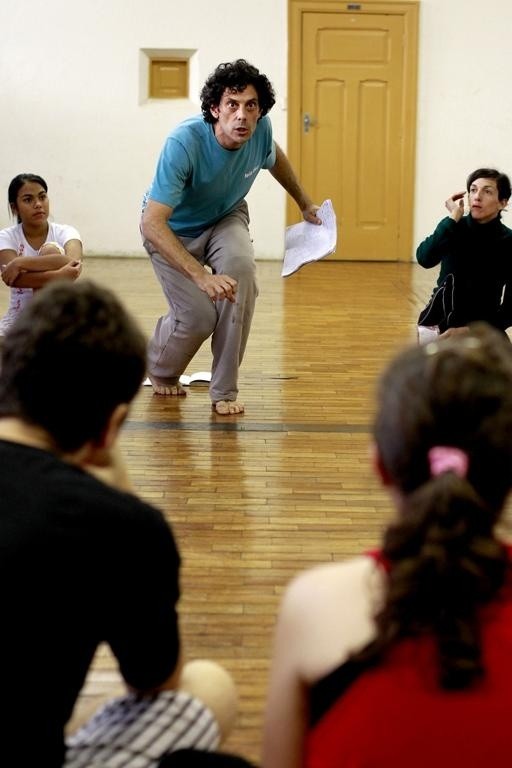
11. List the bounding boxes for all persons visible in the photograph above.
[141,59,323,416]
[0,171,81,346]
[0,277,237,767]
[415,167,511,339]
[258,339,511,768]
